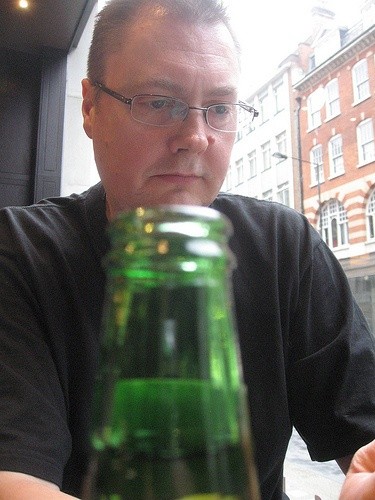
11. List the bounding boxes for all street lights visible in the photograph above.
[272,151,322,241]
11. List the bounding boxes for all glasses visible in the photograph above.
[92,81,260,133]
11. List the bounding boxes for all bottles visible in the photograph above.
[79,205,261,500]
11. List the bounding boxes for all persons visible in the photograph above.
[0,1,375,500]
